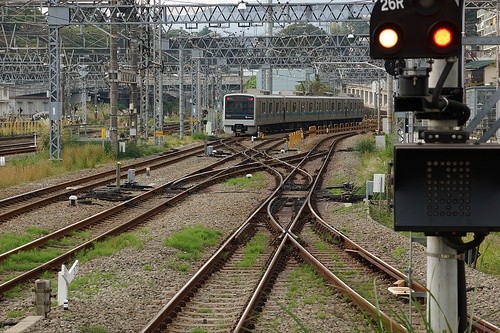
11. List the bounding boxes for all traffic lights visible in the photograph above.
[369,0,461,60]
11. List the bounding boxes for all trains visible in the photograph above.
[222,91,366,136]
